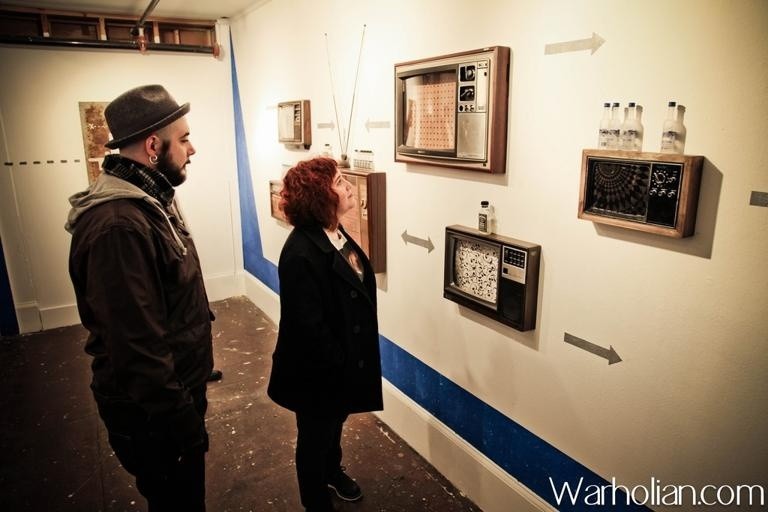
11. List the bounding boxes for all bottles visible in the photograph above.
[478,200,492,235]
[607,102,621,150]
[598,102,610,150]
[622,102,641,150]
[660,102,676,153]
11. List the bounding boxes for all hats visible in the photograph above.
[103,84,190,150]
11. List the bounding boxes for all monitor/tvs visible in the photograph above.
[339,173,370,262]
[271,193,290,222]
[278,101,302,142]
[396,58,490,163]
[444,230,528,324]
[583,156,684,229]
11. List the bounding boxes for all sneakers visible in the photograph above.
[327,470,363,501]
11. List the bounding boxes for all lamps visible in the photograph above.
[137,24,148,52]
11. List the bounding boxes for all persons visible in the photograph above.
[62,85,216,509]
[266,156,385,511]
